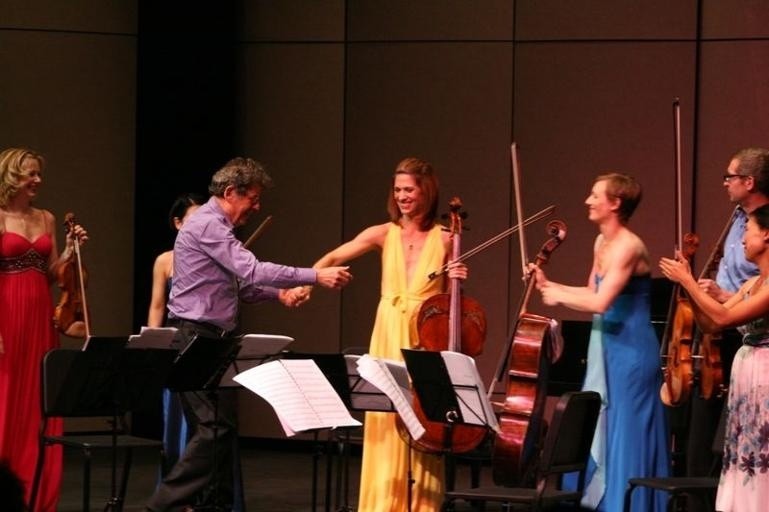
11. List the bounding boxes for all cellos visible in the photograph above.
[395,196,488,452]
[491,219,567,486]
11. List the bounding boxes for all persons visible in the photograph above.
[148,157,353,512]
[0,148,88,512]
[522,173,669,512]
[699,149,769,393]
[297,157,468,512]
[659,204,768,512]
[148,195,248,512]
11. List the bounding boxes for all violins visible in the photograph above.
[702,332,722,400]
[53,211,91,334]
[665,232,701,404]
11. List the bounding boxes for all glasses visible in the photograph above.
[722,175,755,181]
[240,185,260,204]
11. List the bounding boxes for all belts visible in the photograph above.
[188,316,240,340]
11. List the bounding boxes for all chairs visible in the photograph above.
[446,390,602,512]
[28,348,166,512]
[622,397,729,510]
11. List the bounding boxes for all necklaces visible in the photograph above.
[400,221,427,251]
[5,206,34,231]
